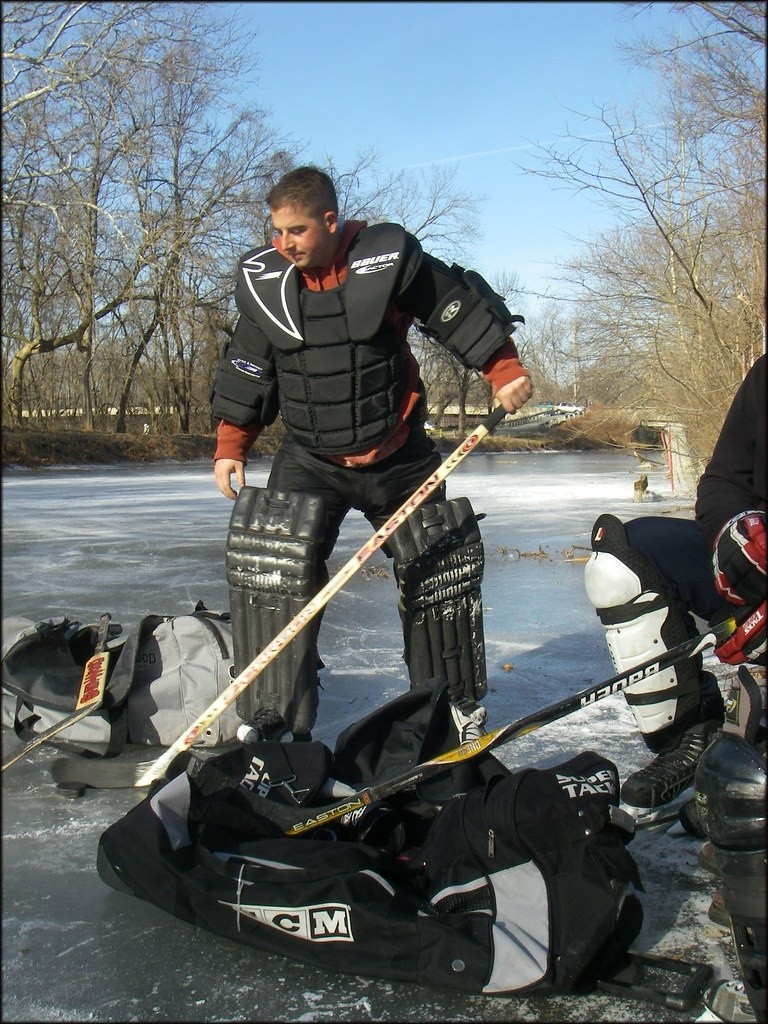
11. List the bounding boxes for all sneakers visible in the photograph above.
[621,728,717,827]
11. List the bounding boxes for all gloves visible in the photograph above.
[713,512,768,606]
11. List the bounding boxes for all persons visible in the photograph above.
[586,355,767,830]
[210,166,533,747]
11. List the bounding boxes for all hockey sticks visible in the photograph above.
[1,610,112,777]
[50,381,536,792]
[163,614,746,840]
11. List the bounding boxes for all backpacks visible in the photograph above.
[96,600,245,753]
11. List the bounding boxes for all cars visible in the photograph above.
[533,400,555,409]
[552,402,585,415]
[423,420,437,431]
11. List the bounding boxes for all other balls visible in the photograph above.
[54,781,87,799]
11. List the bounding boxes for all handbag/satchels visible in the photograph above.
[95,687,641,1000]
